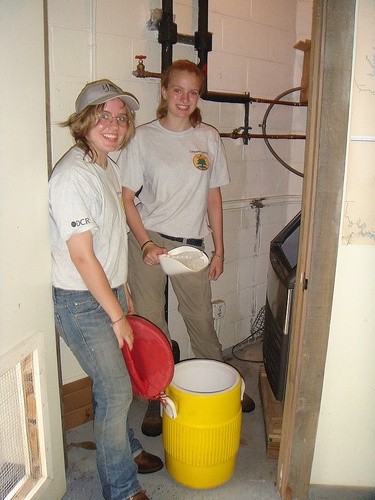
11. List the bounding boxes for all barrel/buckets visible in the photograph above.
[158,358,246,491]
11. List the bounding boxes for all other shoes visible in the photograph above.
[141,405,163,437]
[126,490,149,500]
[134,451,163,473]
[242,393,255,412]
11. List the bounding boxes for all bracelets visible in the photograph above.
[111,309,126,325]
[140,240,153,251]
[212,251,224,261]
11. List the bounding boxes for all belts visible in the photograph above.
[158,233,202,246]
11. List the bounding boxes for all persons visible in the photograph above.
[117,59,256,437]
[47,78,164,500]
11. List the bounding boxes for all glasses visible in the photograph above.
[96,110,129,127]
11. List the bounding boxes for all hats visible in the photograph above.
[75,79,140,114]
